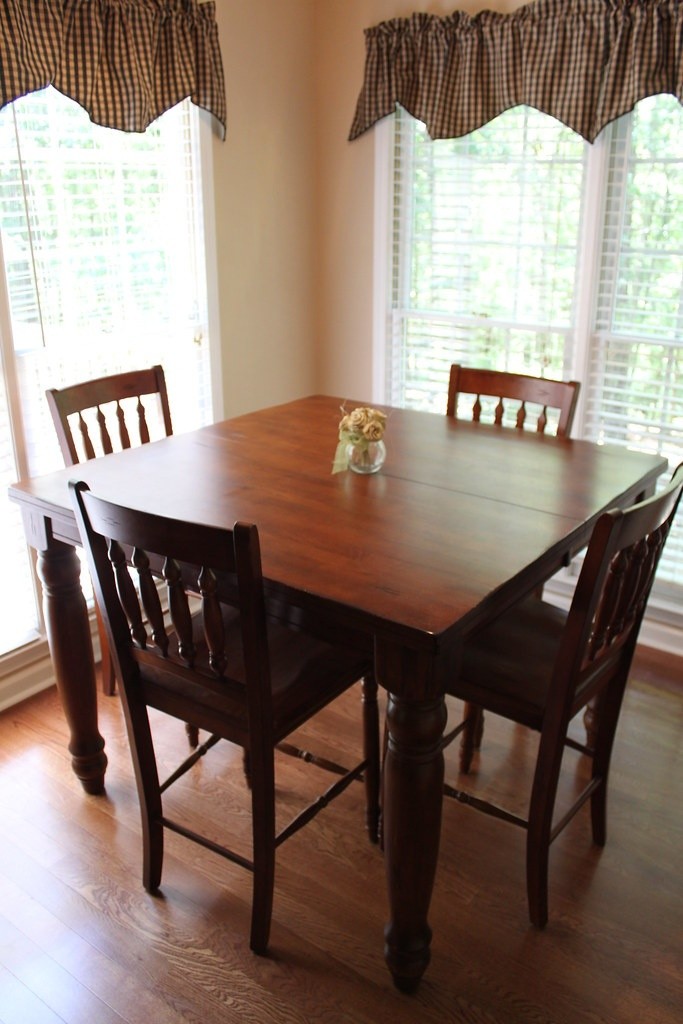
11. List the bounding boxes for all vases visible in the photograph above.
[345,436,387,474]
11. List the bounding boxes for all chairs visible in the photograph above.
[376,460,683,929]
[45,365,203,749]
[68,479,380,956]
[444,364,582,740]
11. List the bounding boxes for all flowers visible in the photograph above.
[331,404,388,474]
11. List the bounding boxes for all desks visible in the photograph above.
[10,394,668,992]
